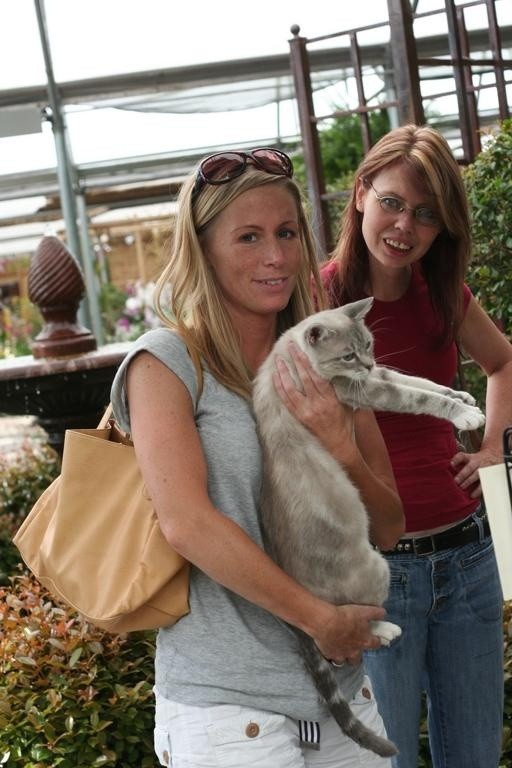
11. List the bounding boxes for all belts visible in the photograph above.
[370,505,494,556]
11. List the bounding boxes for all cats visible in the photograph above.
[250,296,488,759]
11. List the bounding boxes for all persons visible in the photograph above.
[307,121,511,768]
[109,145,409,768]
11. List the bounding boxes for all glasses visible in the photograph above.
[191,146,294,206]
[362,177,444,227]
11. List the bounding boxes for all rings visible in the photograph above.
[331,660,346,668]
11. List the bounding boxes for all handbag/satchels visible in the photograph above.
[8,322,206,636]
